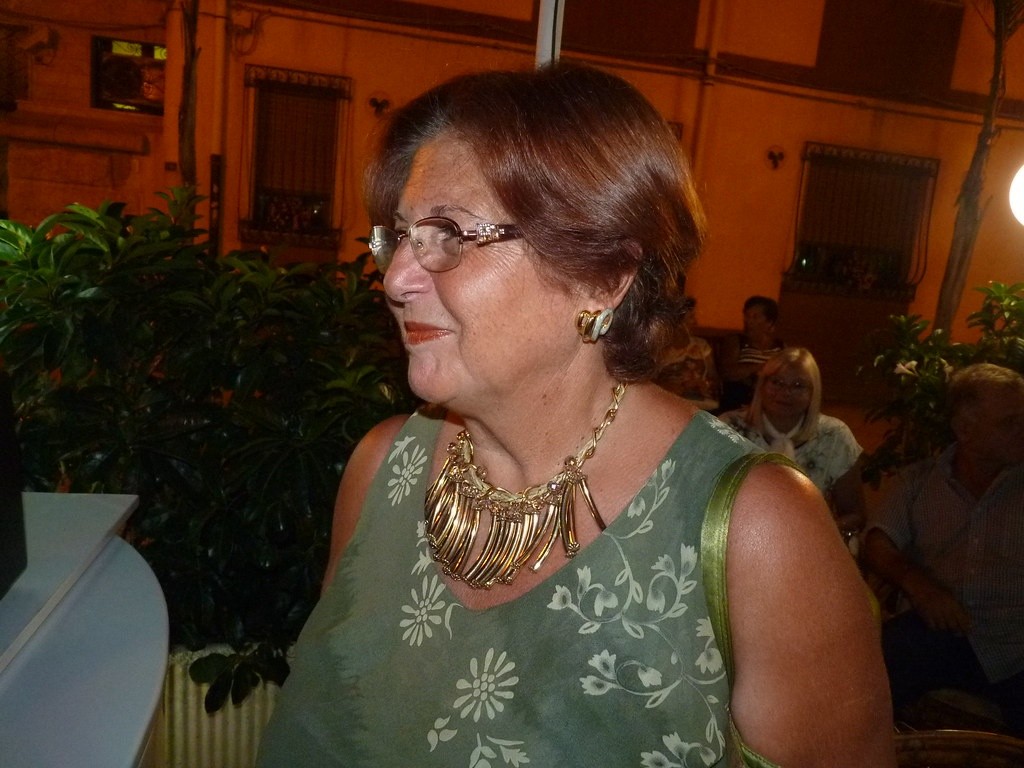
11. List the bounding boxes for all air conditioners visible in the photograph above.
[97,52,165,108]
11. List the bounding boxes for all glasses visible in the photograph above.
[366,214,524,275]
[766,377,812,392]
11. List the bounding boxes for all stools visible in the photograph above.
[887,686,1017,735]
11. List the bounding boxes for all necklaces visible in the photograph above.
[426,380,628,591]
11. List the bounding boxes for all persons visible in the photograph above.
[861,363,1024,740]
[717,296,783,416]
[653,296,718,413]
[255,62,894,768]
[719,349,870,533]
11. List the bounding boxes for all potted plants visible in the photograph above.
[0,182,424,767]
